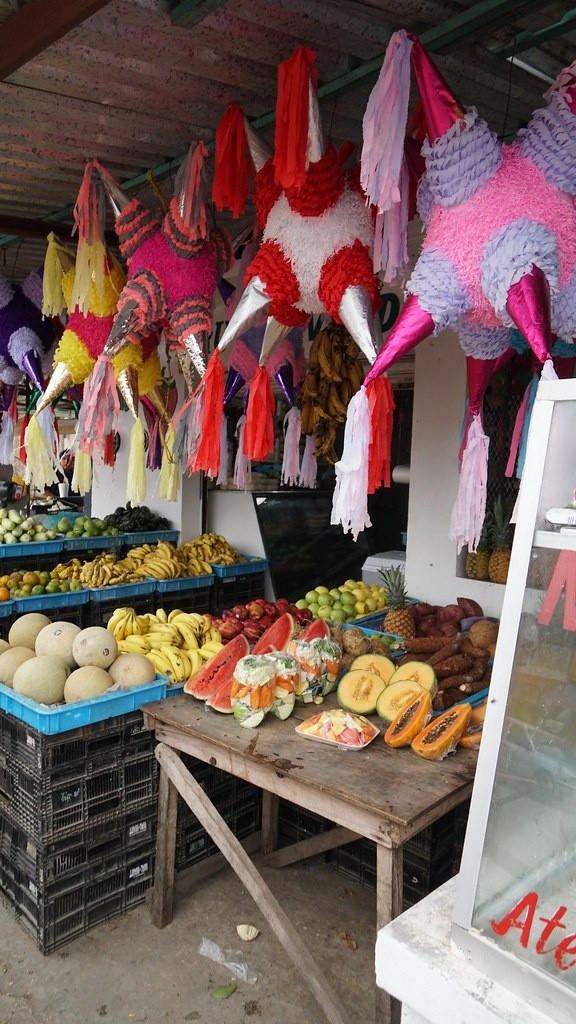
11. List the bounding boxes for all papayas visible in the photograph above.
[384,692,488,761]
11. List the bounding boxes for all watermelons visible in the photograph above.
[183,613,333,715]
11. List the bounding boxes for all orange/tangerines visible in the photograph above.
[0,570,82,601]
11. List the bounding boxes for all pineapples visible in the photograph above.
[375,563,418,639]
[466,501,510,582]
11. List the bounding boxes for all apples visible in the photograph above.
[202,579,388,642]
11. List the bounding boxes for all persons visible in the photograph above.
[45,449,84,512]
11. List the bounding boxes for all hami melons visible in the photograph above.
[336,653,441,722]
[0,613,156,706]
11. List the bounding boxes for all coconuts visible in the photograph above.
[468,621,498,658]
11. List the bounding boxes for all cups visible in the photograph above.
[58,483,70,498]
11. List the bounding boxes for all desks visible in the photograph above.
[136,682,481,1024]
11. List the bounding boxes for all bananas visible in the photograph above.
[106,607,223,683]
[298,320,365,465]
[50,534,246,587]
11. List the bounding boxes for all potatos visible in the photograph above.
[396,636,491,712]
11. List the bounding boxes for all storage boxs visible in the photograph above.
[205,488,373,604]
[0,530,497,959]
[371,377,576,1024]
